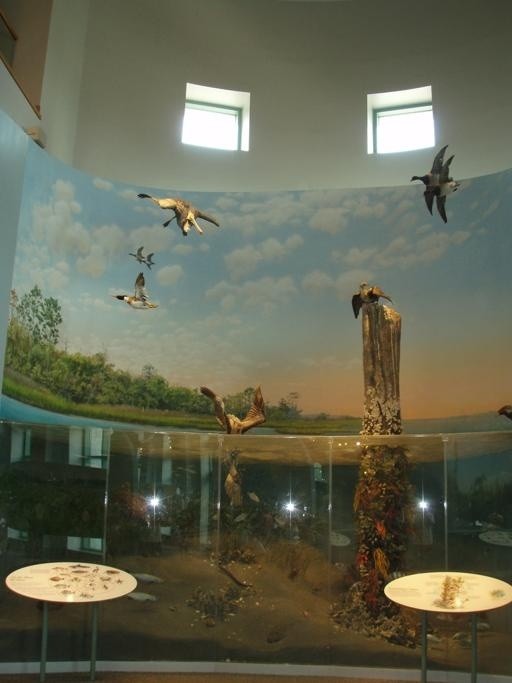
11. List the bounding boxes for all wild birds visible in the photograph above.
[143,252,156,269]
[108,270,161,310]
[435,151,462,223]
[350,281,396,320]
[198,384,268,433]
[135,190,221,237]
[409,144,450,214]
[127,245,147,263]
